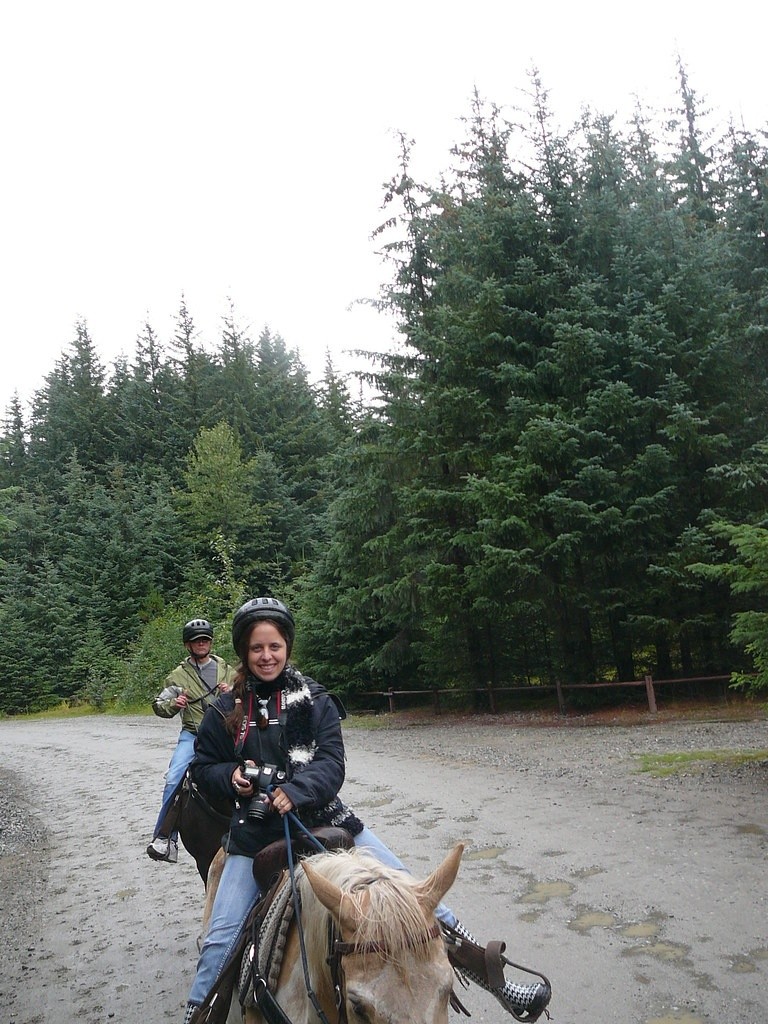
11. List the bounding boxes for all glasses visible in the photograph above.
[190,639,211,642]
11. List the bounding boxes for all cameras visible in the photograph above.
[239,761,289,828]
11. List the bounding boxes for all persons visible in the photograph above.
[185,597,549,1024]
[147,619,240,863]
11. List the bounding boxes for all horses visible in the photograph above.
[203,843,466,1024]
[178,791,232,893]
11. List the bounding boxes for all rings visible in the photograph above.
[279,803,285,808]
[233,781,242,793]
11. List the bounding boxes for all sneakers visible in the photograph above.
[148,838,178,863]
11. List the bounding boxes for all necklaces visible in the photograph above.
[250,685,279,718]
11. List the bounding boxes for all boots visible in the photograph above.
[443,920,549,1015]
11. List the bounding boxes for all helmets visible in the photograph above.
[233,596,294,657]
[182,619,214,641]
